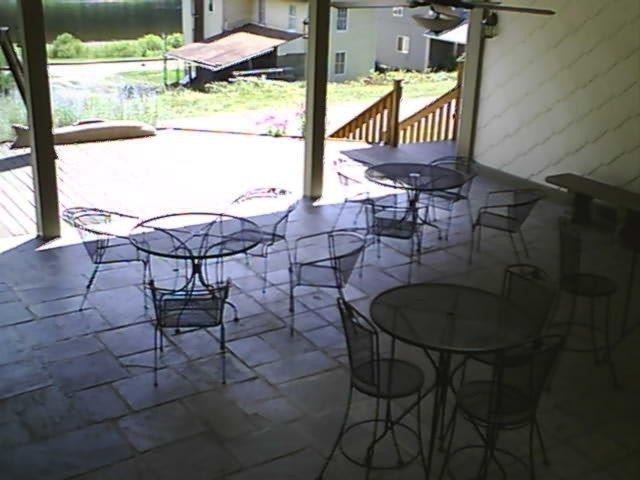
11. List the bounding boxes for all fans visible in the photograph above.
[329,0,555,18]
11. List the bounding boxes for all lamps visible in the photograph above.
[412,6,464,36]
[482,13,498,38]
